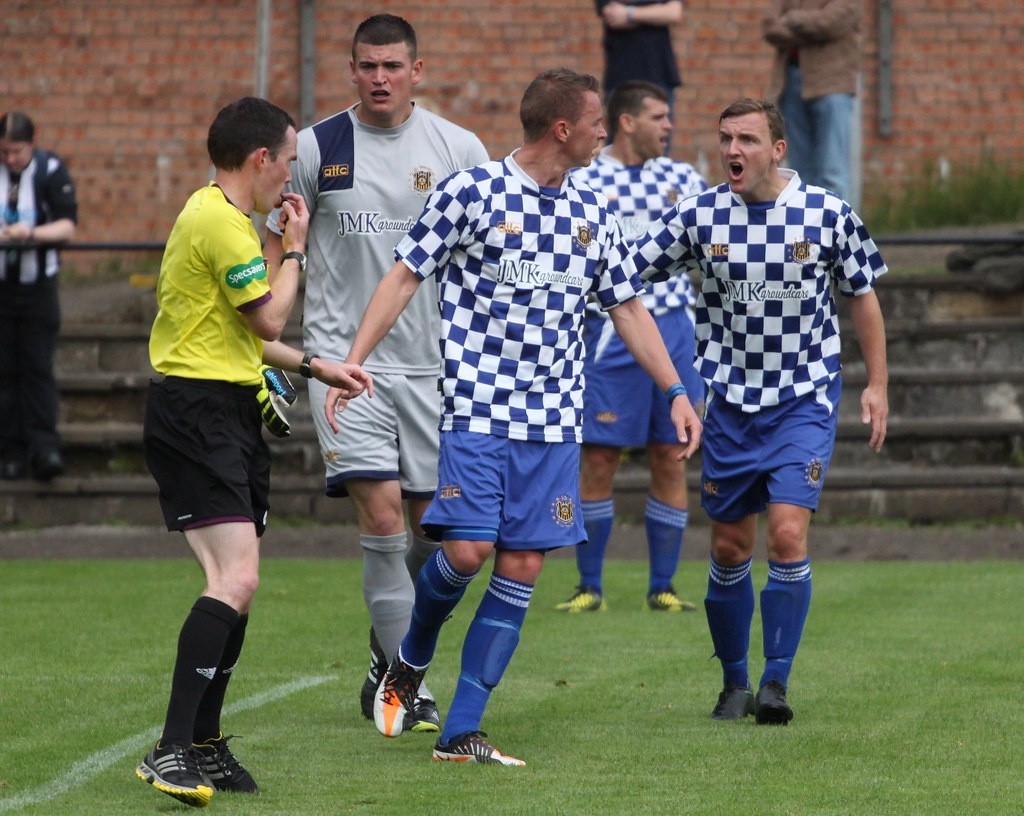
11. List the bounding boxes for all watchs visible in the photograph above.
[281,252,307,271]
[300,351,320,379]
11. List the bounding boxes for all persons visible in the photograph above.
[595,0,683,157]
[0,111,78,479]
[325,70,889,769]
[134,98,374,808]
[263,14,490,729]
[761,0,863,207]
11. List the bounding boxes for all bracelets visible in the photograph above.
[626,6,634,23]
[25,227,35,243]
[665,382,687,404]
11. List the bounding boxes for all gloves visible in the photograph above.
[253,362,303,442]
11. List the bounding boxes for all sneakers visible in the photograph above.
[699,682,800,729]
[430,720,529,778]
[637,586,701,621]
[373,645,432,738]
[130,728,272,806]
[361,626,391,720]
[405,684,441,735]
[548,581,612,618]
